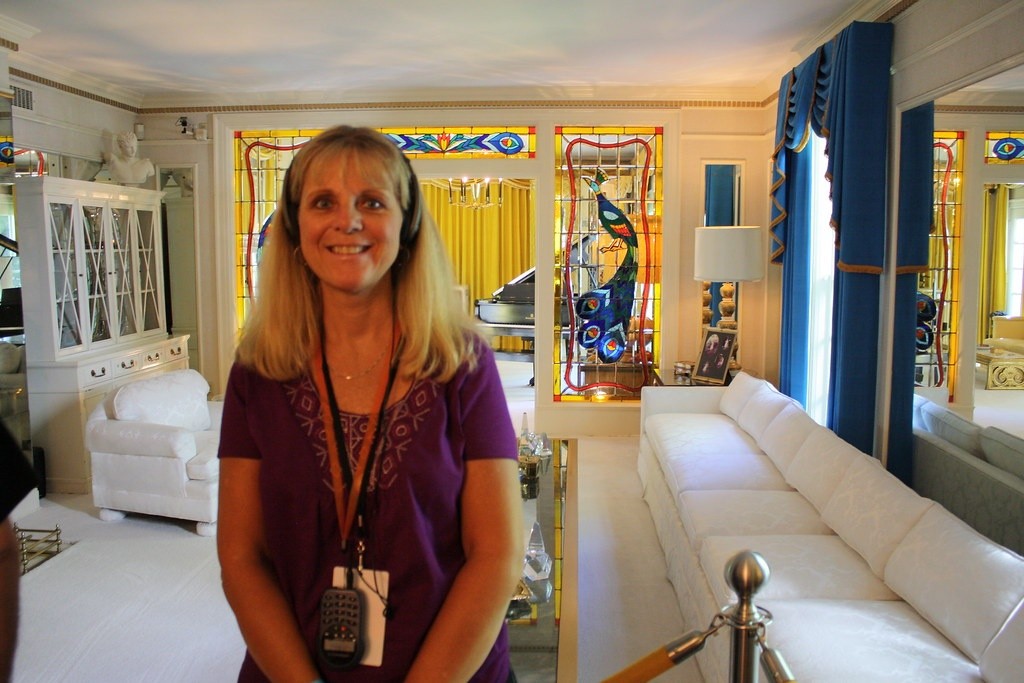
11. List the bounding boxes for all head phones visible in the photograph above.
[281,132,422,249]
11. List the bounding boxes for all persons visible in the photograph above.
[217,124,525,683]
[105,131,155,187]
[0,417,38,683]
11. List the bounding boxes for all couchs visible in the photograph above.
[984,314,1023,355]
[637,374,1024,682]
[86,370,226,535]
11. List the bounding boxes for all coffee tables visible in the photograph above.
[503,436,563,628]
[976,349,1024,391]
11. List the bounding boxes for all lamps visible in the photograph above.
[694,227,763,381]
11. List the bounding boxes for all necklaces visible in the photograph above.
[328,344,388,380]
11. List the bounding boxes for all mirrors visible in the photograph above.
[156,164,201,374]
[883,57,1024,495]
[700,159,743,371]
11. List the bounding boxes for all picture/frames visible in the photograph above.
[693,330,740,384]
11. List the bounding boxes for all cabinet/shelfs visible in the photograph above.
[16,177,192,494]
